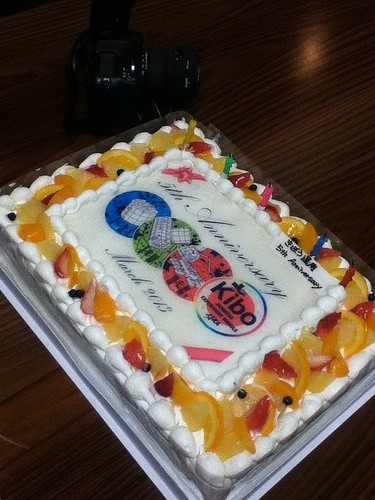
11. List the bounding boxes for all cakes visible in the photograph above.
[0,119,375,500]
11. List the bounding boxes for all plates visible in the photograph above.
[1,273,374,500]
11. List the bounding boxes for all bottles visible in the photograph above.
[66,1,143,133]
[144,47,202,102]
